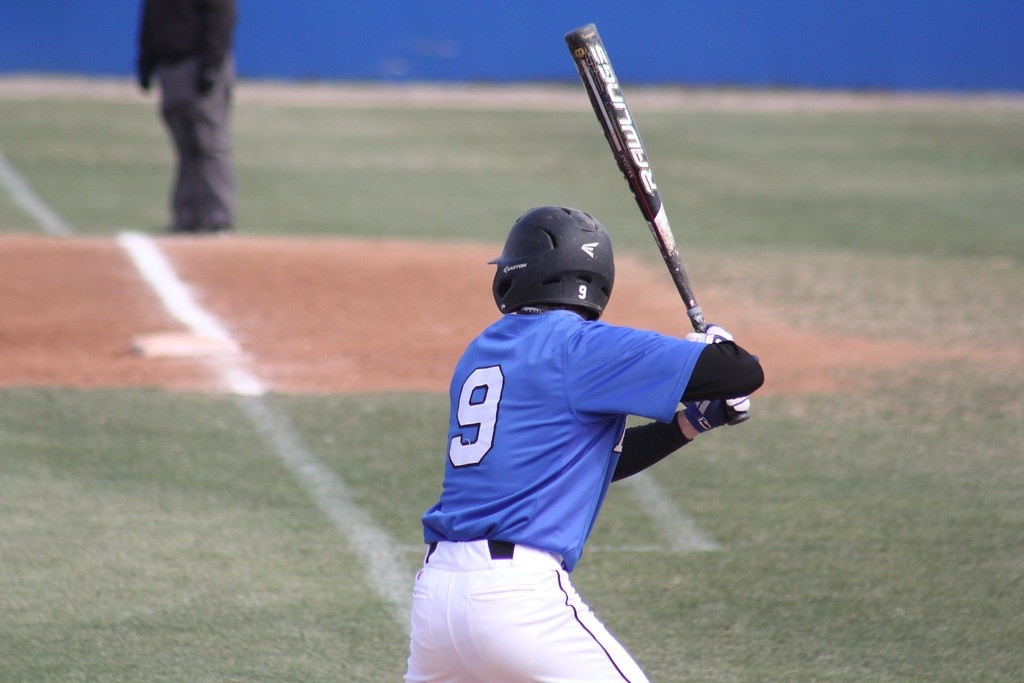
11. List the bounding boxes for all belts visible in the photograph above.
[425,539,515,562]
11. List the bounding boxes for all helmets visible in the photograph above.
[486,207,615,316]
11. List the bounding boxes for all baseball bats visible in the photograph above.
[562,21,750,427]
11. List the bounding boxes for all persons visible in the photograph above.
[138,0,237,232]
[404,206,765,683]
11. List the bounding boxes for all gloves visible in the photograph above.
[683,322,750,432]
[138,60,152,89]
[196,67,216,94]
